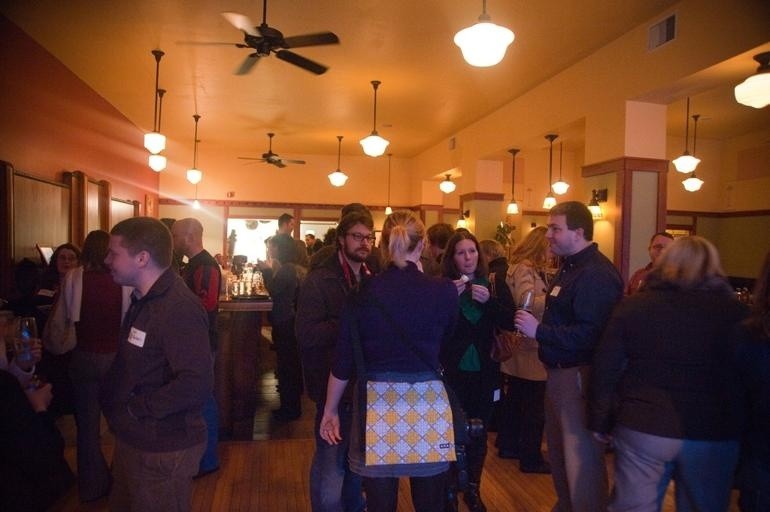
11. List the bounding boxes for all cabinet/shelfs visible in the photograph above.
[735,288,742,303]
[742,287,750,304]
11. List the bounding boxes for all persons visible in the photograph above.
[439,229,516,508]
[294,211,377,508]
[514,202,622,510]
[585,237,756,509]
[98,217,214,508]
[171,217,221,477]
[319,210,460,510]
[496,225,551,475]
[260,202,516,420]
[2,227,138,509]
[625,233,674,296]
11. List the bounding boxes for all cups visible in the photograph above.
[345,232,376,244]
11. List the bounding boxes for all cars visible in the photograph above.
[22,374,48,392]
[226,263,265,295]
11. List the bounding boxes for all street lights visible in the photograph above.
[490,330,523,362]
[360,374,458,467]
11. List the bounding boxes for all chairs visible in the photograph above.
[521,463,551,473]
[499,446,518,459]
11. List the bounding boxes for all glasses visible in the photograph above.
[551,142,571,195]
[144,50,167,155]
[148,89,167,172]
[327,136,349,187]
[542,134,559,209]
[671,97,701,173]
[456,210,470,229]
[439,174,456,194]
[734,50,770,109]
[681,114,704,193]
[385,154,393,215]
[359,80,390,157]
[193,140,201,210]
[453,0,516,67]
[506,148,521,214]
[186,114,202,184]
[586,189,608,219]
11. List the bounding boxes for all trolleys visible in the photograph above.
[238,133,306,169]
[174,0,341,76]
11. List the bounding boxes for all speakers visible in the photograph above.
[463,430,487,512]
[443,444,466,512]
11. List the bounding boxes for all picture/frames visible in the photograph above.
[217,290,280,434]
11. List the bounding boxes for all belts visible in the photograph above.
[512,288,536,337]
[12,317,38,367]
